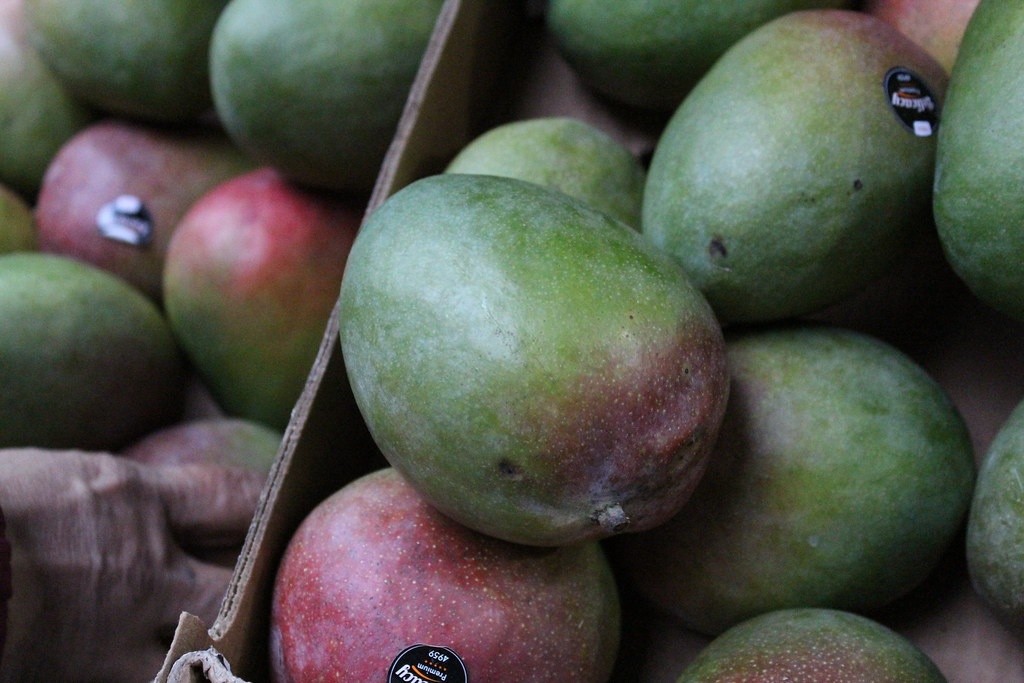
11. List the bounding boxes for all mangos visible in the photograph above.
[0,0,1024,683]
